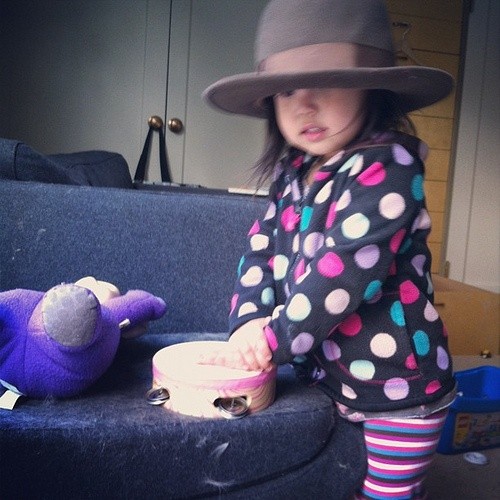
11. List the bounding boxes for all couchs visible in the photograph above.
[0,139,367,500]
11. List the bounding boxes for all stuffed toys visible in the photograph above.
[0,276,167,411]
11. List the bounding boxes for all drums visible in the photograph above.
[144,339,278,422]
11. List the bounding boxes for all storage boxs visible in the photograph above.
[432,272,500,456]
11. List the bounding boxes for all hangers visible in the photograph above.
[392,20,427,67]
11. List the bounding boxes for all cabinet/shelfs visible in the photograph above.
[0,1,266,193]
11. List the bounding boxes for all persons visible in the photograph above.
[201,0,457,500]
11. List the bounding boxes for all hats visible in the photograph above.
[201,0,456,119]
